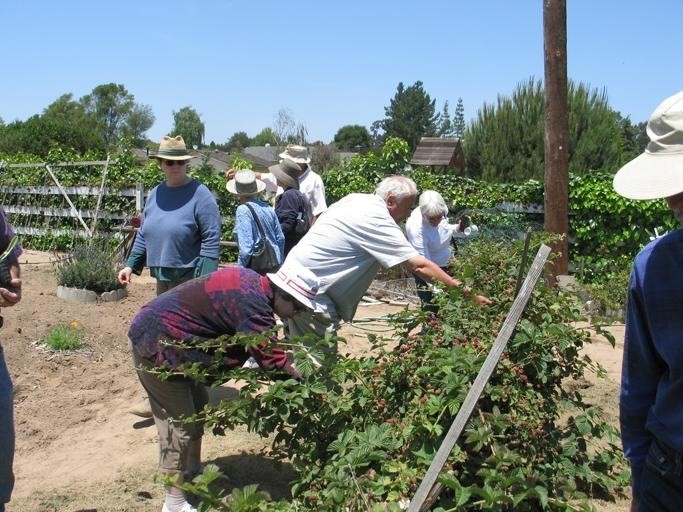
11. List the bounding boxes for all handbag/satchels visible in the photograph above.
[236,203,279,273]
[277,190,309,241]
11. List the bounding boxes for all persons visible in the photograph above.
[268,158,312,254]
[225,169,285,275]
[128,261,319,511]
[119,134,220,417]
[0,199,23,511]
[612,88,683,510]
[226,142,327,225]
[281,174,494,369]
[405,189,470,312]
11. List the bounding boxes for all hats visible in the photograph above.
[226,169,266,195]
[613,92,683,199]
[266,262,321,310]
[269,160,302,190]
[279,144,311,164]
[149,136,196,160]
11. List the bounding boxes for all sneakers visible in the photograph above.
[128,399,153,418]
[162,501,196,512]
